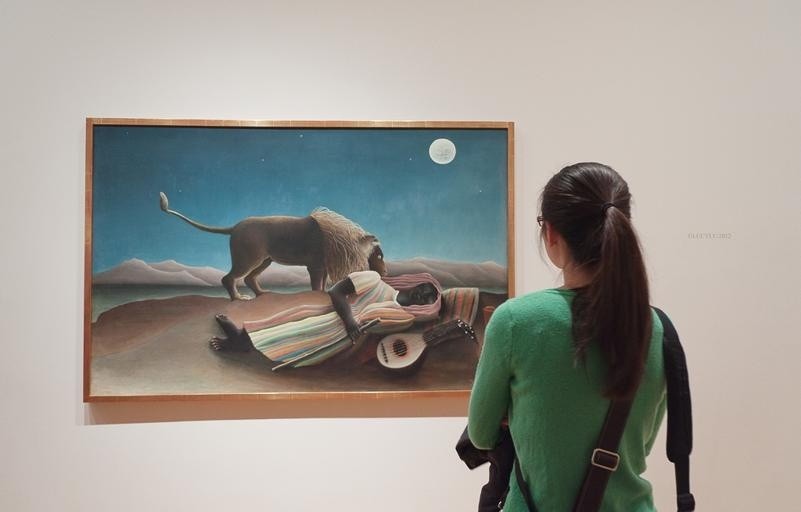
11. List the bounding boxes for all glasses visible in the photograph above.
[536,215,546,227]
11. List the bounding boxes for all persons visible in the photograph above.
[467,161,677,510]
[209,268,444,376]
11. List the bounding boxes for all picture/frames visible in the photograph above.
[82,120,515,403]
